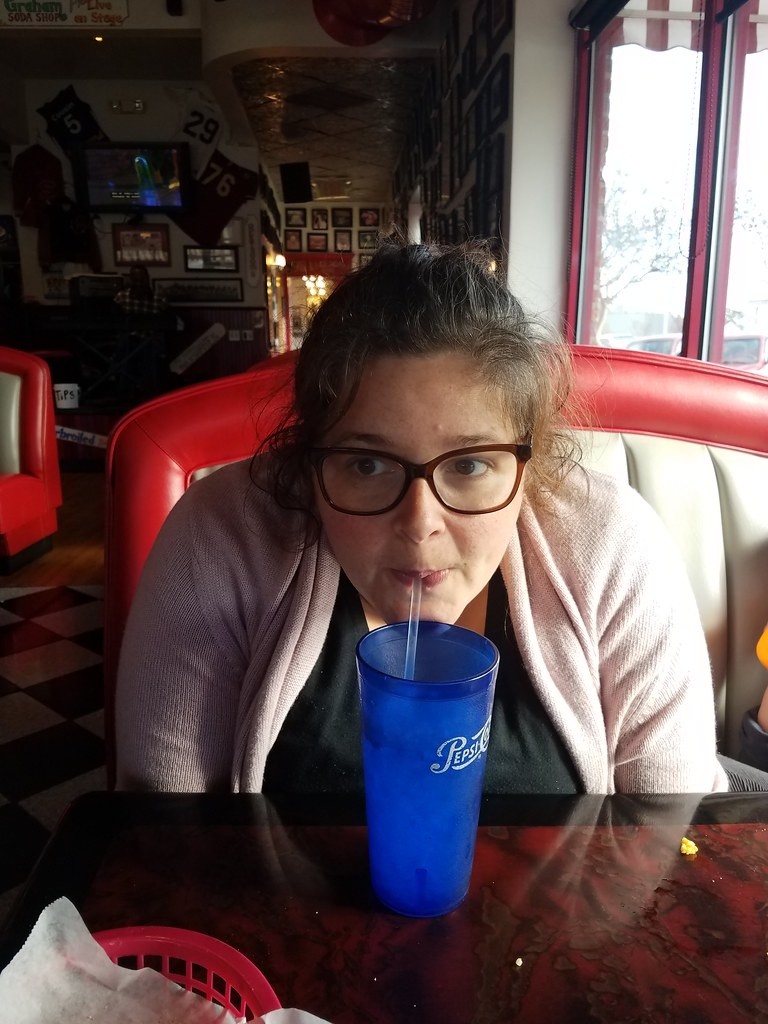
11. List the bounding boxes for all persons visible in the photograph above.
[113,258,169,317]
[105,227,736,794]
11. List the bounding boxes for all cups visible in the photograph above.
[53,383,78,409]
[356,621,500,917]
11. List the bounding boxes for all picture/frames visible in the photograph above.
[331,208,352,228]
[359,231,378,250]
[359,253,373,272]
[335,229,352,253]
[359,209,379,228]
[151,279,243,303]
[285,229,302,253]
[111,223,171,268]
[307,233,327,252]
[312,209,328,231]
[184,246,239,274]
[391,0,513,254]
[286,208,306,228]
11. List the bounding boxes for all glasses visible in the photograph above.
[304,427,534,517]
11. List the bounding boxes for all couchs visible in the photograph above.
[103,343,768,797]
[0,347,64,578]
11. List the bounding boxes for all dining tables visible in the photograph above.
[0,790,768,1024]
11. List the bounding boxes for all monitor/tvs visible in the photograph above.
[76,142,193,214]
